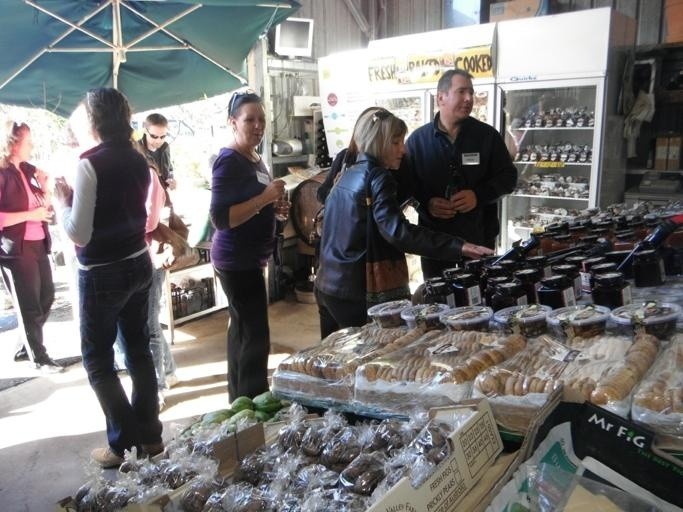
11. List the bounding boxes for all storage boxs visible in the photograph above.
[142,397,505,511]
[488,0,549,24]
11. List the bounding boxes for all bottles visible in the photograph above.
[316,118,332,168]
[274,188,290,220]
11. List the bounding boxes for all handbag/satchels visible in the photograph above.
[144,222,200,271]
[168,212,189,240]
[364,250,412,323]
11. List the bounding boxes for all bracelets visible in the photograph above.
[251,196,259,214]
[461,245,464,258]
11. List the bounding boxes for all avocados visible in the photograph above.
[195,390,289,439]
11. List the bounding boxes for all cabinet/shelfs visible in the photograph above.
[480,0,683,206]
[156,249,268,345]
[625,42,683,174]
[254,37,326,304]
[365,7,638,280]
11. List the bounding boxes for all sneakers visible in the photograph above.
[138,442,164,457]
[88,441,147,468]
[164,367,181,387]
[33,356,64,373]
[156,378,169,411]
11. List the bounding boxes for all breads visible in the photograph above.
[59,440,223,512]
[355,329,528,388]
[471,333,663,406]
[277,325,426,380]
[223,411,461,512]
[633,332,682,418]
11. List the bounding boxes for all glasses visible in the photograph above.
[230,88,255,117]
[145,128,169,140]
[368,110,393,130]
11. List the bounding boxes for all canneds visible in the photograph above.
[418,214,682,308]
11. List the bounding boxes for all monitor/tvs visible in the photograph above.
[273,17,314,63]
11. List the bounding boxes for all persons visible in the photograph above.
[55,87,165,468]
[404,69,518,282]
[132,112,180,389]
[316,107,410,209]
[208,90,292,402]
[314,110,494,338]
[1,119,64,375]
